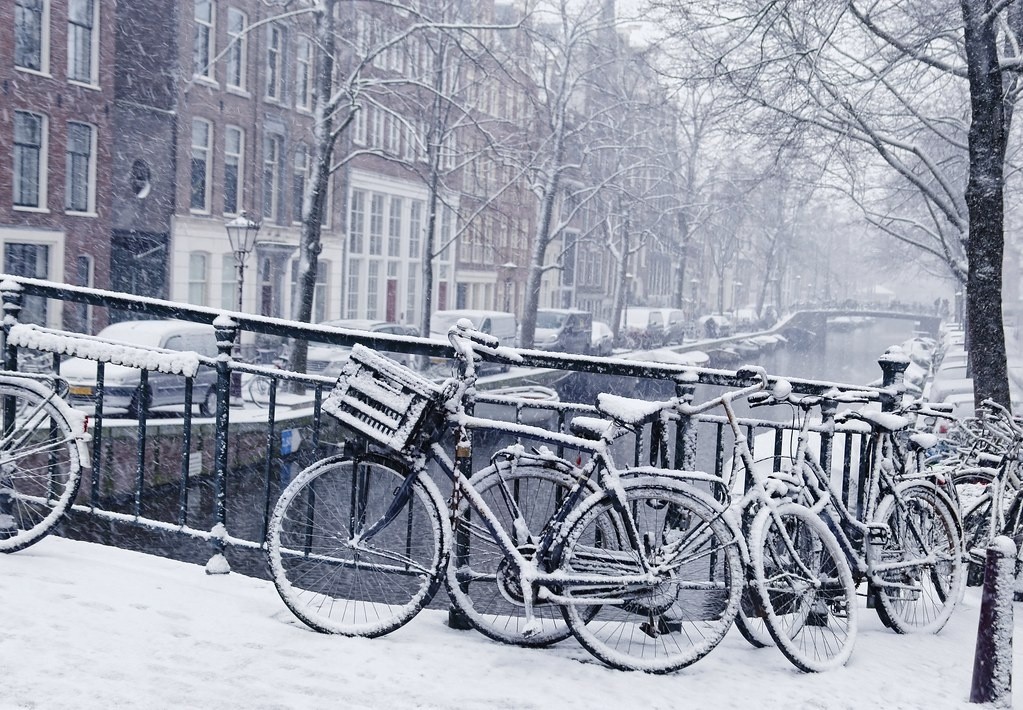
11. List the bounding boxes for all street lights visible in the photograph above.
[735,283,742,312]
[224,210,259,406]
[691,279,700,323]
[626,273,633,328]
[807,284,811,306]
[797,276,801,309]
[769,279,778,305]
[503,261,516,310]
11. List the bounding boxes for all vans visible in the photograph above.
[427,311,517,372]
[514,308,592,357]
[620,307,684,344]
[58,320,219,418]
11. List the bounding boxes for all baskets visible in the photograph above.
[958,417,1014,477]
[321,343,441,456]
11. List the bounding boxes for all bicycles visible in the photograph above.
[232,352,276,408]
[722,378,1023,649]
[0,361,94,553]
[268,308,751,675]
[447,364,857,672]
[617,325,654,350]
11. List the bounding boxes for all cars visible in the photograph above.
[749,305,778,330]
[713,312,737,334]
[306,318,412,381]
[700,315,731,338]
[734,309,759,332]
[923,322,1023,438]
[590,321,614,357]
